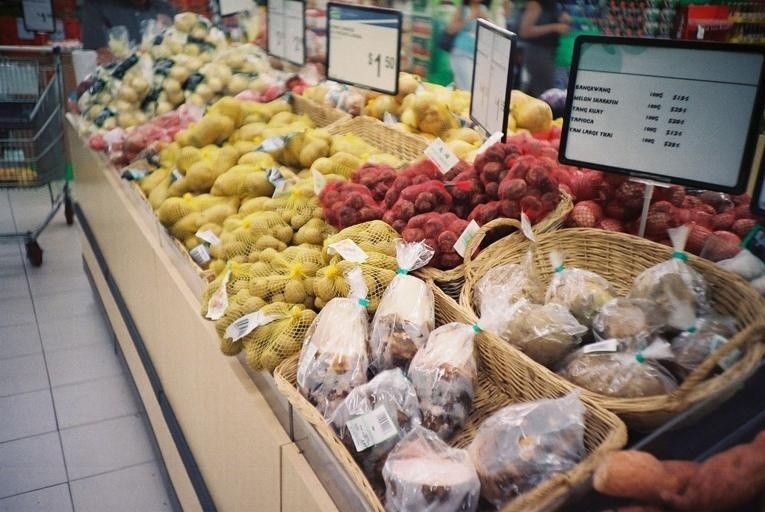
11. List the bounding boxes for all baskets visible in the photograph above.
[120,92,765,512]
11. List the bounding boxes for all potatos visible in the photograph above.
[592,431,765,512]
[77,11,758,376]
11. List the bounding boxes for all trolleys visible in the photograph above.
[0,43,78,268]
